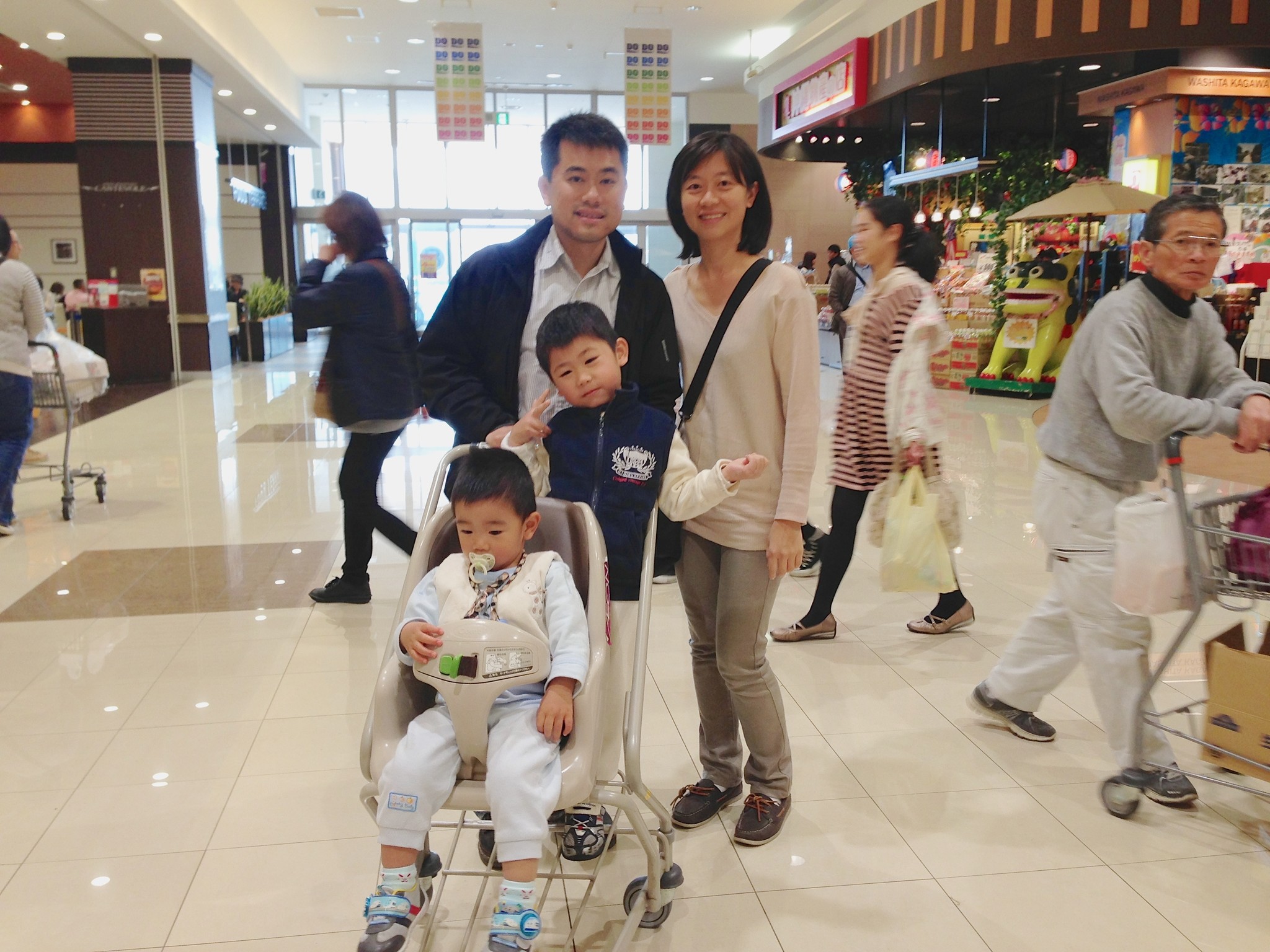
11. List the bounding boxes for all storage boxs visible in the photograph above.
[931,268,996,390]
[1201,623,1270,783]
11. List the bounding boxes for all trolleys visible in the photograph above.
[1098,428,1269,819]
[26,340,111,522]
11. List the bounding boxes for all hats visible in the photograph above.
[848,236,855,249]
[230,274,243,284]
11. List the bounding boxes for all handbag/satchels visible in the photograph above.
[867,470,964,549]
[1113,482,1221,617]
[881,464,958,593]
[652,507,682,585]
[314,365,338,421]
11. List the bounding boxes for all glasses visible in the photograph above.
[1154,235,1229,258]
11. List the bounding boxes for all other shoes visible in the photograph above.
[0,525,15,533]
[770,613,836,642]
[604,810,617,849]
[478,813,503,871]
[907,599,975,634]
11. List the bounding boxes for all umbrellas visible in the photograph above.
[1004,176,1166,318]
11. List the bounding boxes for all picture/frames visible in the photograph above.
[50,237,78,265]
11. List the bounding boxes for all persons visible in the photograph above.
[661,133,821,845]
[0,219,92,537]
[966,194,1270,803]
[825,244,846,284]
[795,251,818,285]
[424,112,682,871]
[652,506,827,585]
[224,273,250,358]
[288,191,421,601]
[827,233,874,376]
[501,300,768,861]
[771,191,977,641]
[355,443,590,952]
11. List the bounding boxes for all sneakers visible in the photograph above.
[670,779,743,828]
[561,803,606,861]
[734,793,791,845]
[790,536,822,577]
[1140,762,1198,803]
[358,874,429,952]
[484,901,542,952]
[308,573,372,604]
[965,678,1057,741]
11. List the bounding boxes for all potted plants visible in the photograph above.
[237,273,295,362]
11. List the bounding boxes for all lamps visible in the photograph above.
[914,180,926,223]
[969,172,982,219]
[930,177,943,221]
[949,176,963,220]
[747,30,756,79]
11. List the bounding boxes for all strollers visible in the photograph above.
[358,441,688,952]
